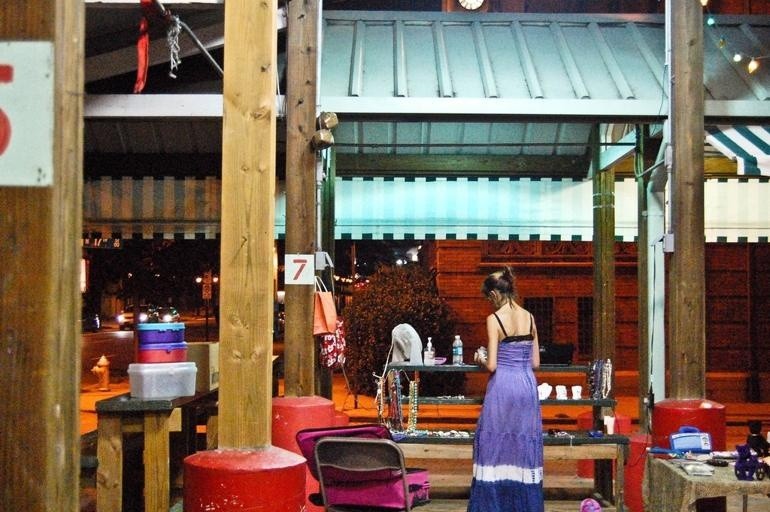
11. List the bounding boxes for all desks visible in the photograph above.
[641,453,770,512]
[95,356,280,512]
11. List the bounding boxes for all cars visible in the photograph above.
[155,306,181,323]
[80,309,100,333]
[273,304,284,342]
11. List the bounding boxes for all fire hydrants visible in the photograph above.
[90,353,112,394]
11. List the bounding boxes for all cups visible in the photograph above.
[557,385,582,400]
[604,416,615,435]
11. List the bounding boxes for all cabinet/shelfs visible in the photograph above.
[389,363,630,512]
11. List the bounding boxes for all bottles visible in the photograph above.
[423,337,435,367]
[452,335,463,366]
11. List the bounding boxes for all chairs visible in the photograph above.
[314,437,429,512]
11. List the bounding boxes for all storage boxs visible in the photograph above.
[187,342,220,393]
[127,323,197,399]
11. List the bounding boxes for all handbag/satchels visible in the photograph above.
[313,276,346,369]
[669,426,712,453]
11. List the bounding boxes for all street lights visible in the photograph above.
[195,273,218,343]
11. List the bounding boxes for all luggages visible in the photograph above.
[296,424,432,509]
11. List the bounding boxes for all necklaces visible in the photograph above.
[376,368,420,435]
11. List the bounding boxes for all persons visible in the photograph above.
[468,266,544,512]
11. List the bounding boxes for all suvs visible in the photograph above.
[117,304,159,330]
